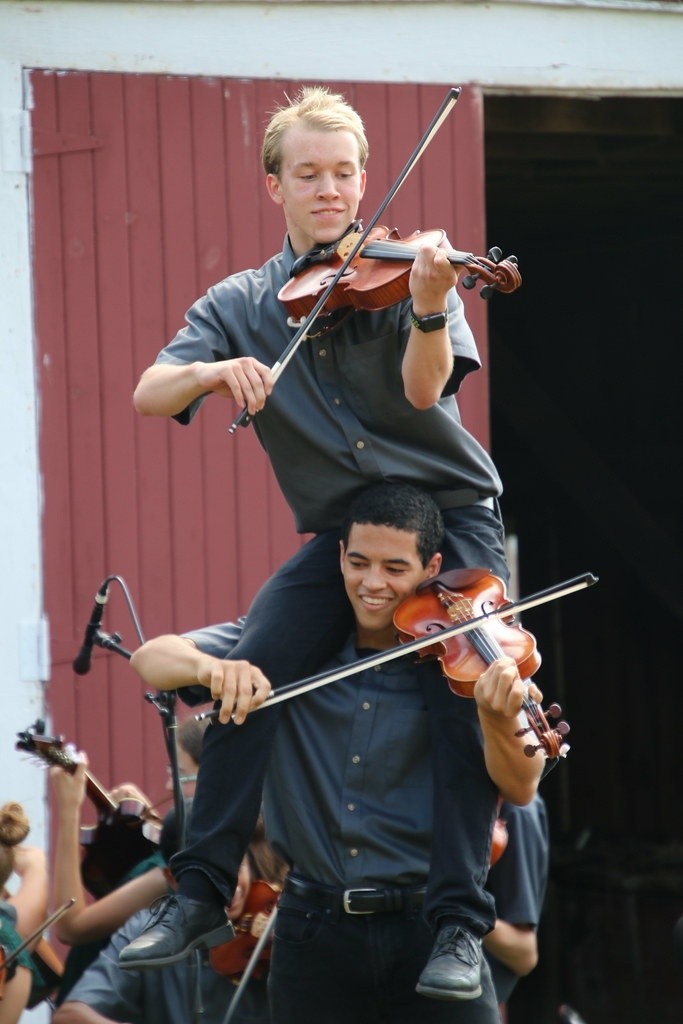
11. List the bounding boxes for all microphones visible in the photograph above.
[72,582,109,675]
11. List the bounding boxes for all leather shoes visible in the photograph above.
[415,924,484,1000]
[118,894,236,967]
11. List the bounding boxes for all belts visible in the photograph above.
[276,876,428,915]
[429,487,494,512]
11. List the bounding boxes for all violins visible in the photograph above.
[277,218,525,326]
[209,879,278,980]
[12,723,175,898]
[394,565,570,762]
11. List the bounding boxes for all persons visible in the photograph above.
[0,712,290,1024]
[481,792,549,1024]
[129,483,546,1024]
[118,89,506,999]
